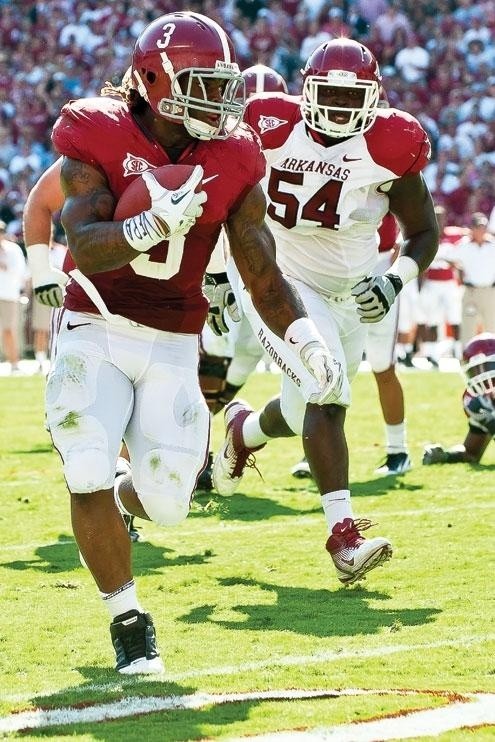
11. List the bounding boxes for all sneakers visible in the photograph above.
[291,456,312,479]
[375,452,411,476]
[197,455,213,489]
[211,399,268,498]
[326,518,393,586]
[110,609,165,675]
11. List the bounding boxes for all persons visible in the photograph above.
[1,2,495,499]
[39,9,347,678]
[210,37,441,589]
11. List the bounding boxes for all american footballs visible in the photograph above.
[113,164,203,223]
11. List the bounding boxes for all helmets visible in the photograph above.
[299,38,389,139]
[121,11,289,141]
[463,332,495,399]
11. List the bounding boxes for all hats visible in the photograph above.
[472,211,490,228]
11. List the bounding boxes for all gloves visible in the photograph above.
[300,341,344,406]
[423,447,447,465]
[142,165,208,237]
[32,267,68,308]
[350,273,404,323]
[205,272,240,336]
